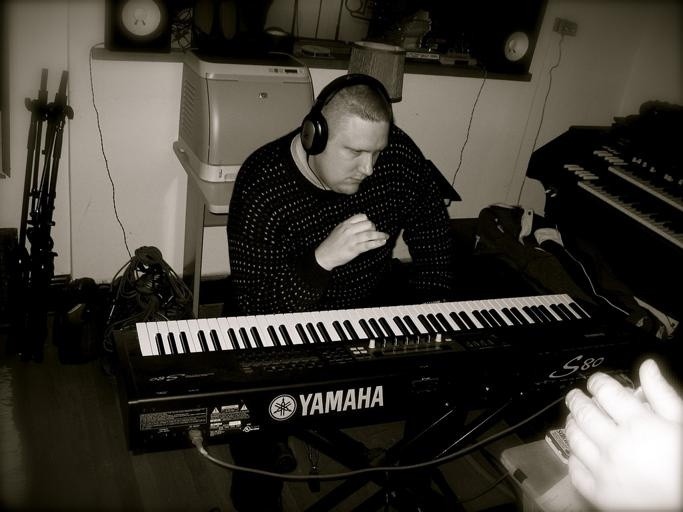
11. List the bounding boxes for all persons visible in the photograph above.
[563,358,683,511]
[226,84,455,512]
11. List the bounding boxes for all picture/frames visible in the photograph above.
[499,427,682,509]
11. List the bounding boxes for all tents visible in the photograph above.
[301,73,395,156]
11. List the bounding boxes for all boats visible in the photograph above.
[482,13,540,75]
[105,0,173,54]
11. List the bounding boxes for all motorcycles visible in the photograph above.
[9,67,74,366]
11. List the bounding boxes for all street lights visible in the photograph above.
[53,277,106,365]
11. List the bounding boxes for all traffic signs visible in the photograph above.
[553,17,577,38]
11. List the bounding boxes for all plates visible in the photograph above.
[171,140,235,319]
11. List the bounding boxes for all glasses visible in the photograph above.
[345,39,407,106]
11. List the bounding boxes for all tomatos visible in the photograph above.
[573,174,683,267]
[110,283,621,452]
[524,101,682,309]
[601,161,683,222]
